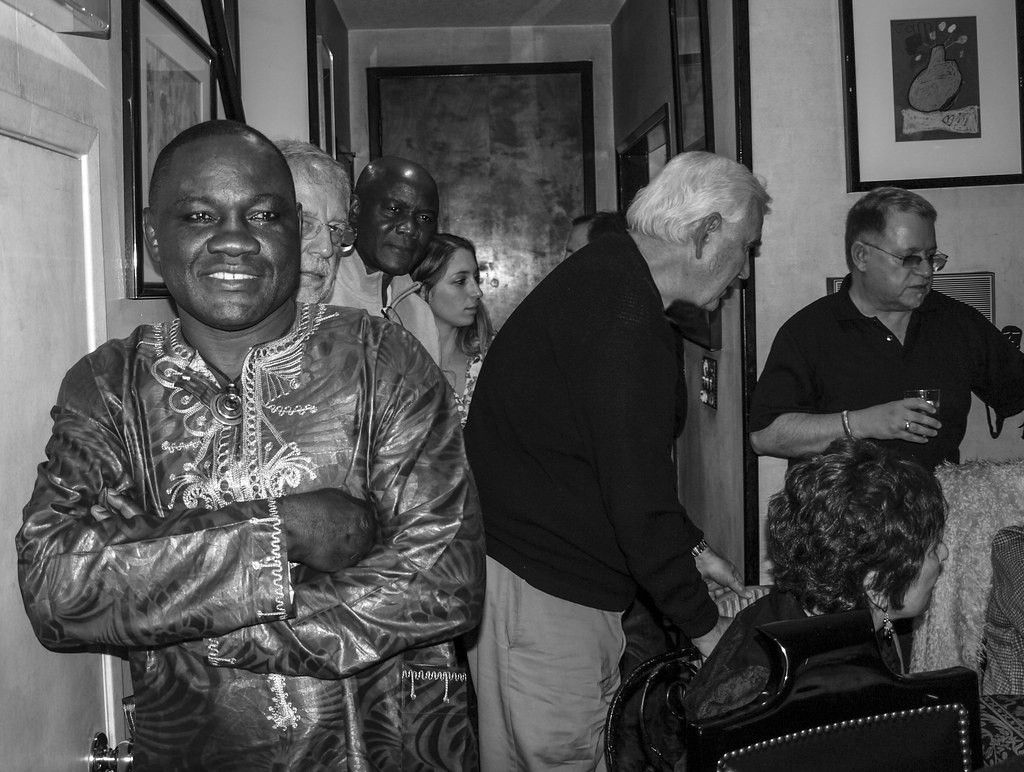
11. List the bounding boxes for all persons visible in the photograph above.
[463,152,771,772]
[564,212,627,260]
[749,186,1024,467]
[411,233,498,427]
[272,139,358,304]
[15,120,487,772]
[325,156,442,366]
[685,437,949,723]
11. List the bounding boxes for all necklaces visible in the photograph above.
[181,327,243,426]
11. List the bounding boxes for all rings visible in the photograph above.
[905,421,910,430]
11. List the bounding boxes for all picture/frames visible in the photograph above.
[120,0,220,301]
[668,0,716,157]
[838,0,1024,192]
[317,34,337,162]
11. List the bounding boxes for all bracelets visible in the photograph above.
[841,410,855,439]
[691,539,709,557]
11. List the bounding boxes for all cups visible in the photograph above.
[902,389,942,446]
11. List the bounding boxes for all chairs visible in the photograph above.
[604,458,1024,772]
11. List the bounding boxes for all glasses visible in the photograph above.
[300,215,357,248]
[861,240,948,274]
